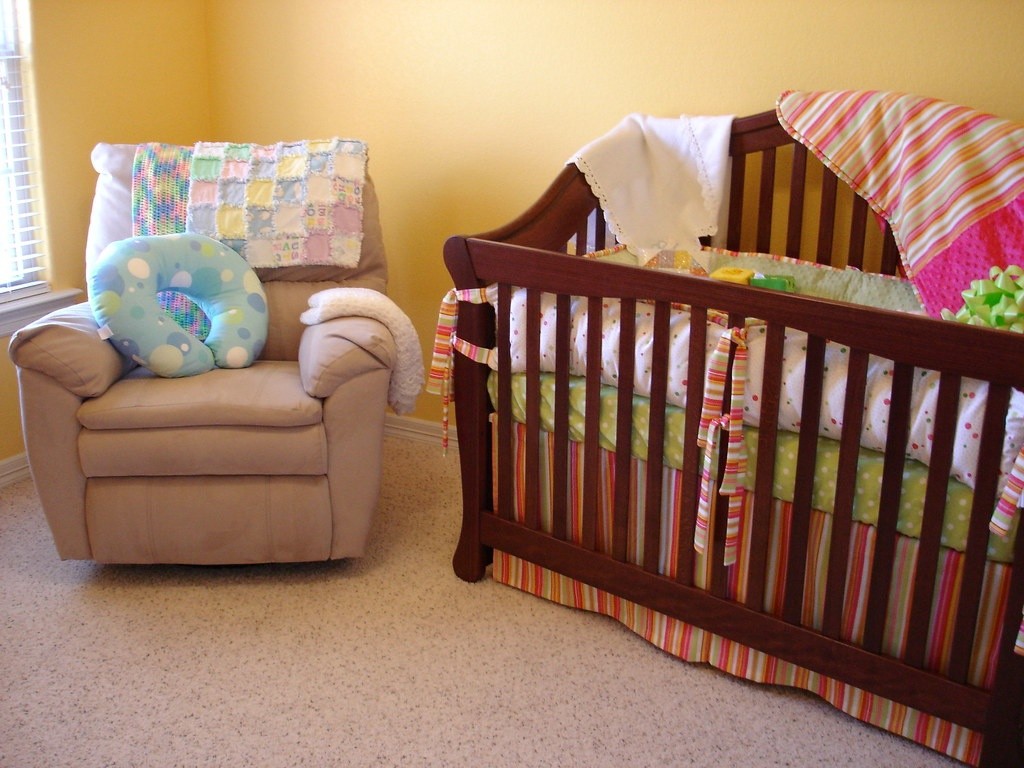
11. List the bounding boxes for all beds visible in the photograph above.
[427,89,1024,767]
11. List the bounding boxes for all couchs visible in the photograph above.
[10,143,397,564]
